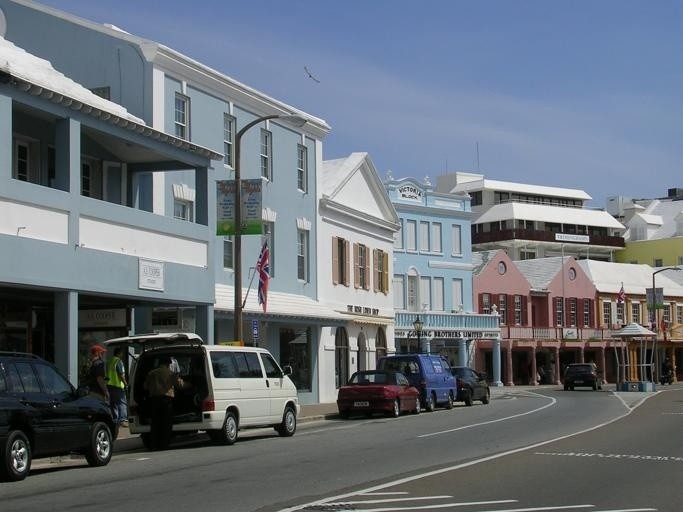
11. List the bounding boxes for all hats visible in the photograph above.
[91,345,108,353]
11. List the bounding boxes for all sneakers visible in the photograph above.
[121,421,128,427]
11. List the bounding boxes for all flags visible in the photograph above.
[256,238,270,314]
[618,288,625,303]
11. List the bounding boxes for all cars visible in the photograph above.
[563,362,604,391]
[445,366,492,407]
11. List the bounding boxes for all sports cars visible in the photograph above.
[335,368,422,416]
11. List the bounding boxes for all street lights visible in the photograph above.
[413,312,425,355]
[232,109,336,353]
[652,267,683,385]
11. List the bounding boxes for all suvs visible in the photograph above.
[0,350,120,483]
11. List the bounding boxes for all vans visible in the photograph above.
[376,350,458,412]
[102,330,303,447]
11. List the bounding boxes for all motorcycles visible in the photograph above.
[659,365,677,385]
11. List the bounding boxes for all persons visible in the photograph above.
[87,345,129,428]
[142,357,184,448]
